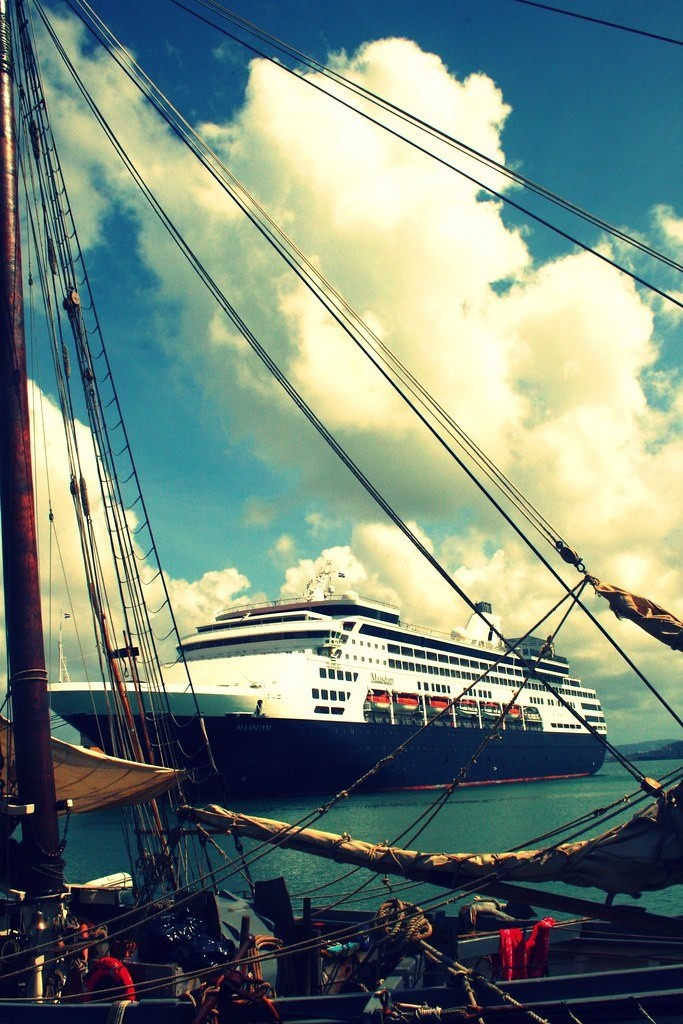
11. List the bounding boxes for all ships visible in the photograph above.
[49,563,607,796]
